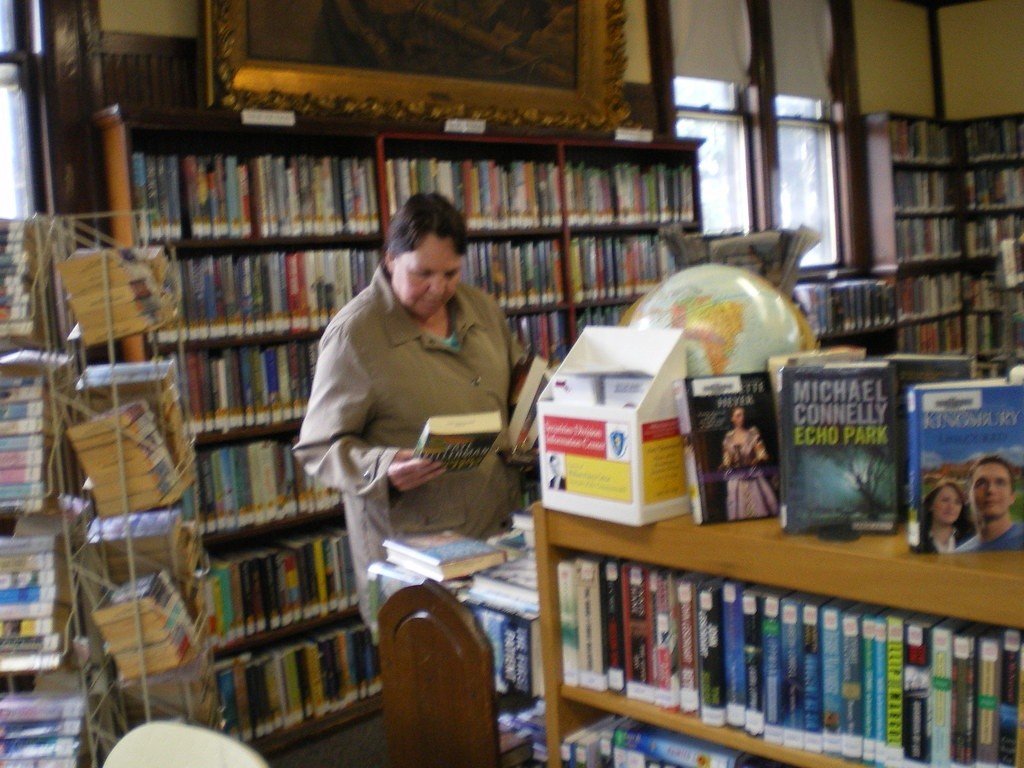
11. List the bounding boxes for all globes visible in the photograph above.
[618,262,816,379]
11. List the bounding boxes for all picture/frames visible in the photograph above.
[199,0,631,139]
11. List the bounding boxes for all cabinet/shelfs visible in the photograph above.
[0,100,1024,768]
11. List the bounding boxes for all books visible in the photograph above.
[0,143,692,768]
[778,360,901,536]
[907,381,1024,551]
[693,117,1024,405]
[886,353,976,523]
[551,549,1024,768]
[672,370,782,526]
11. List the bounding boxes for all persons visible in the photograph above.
[911,453,1024,552]
[718,407,779,521]
[546,453,566,491]
[293,193,533,636]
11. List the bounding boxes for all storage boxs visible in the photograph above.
[537,324,693,524]
